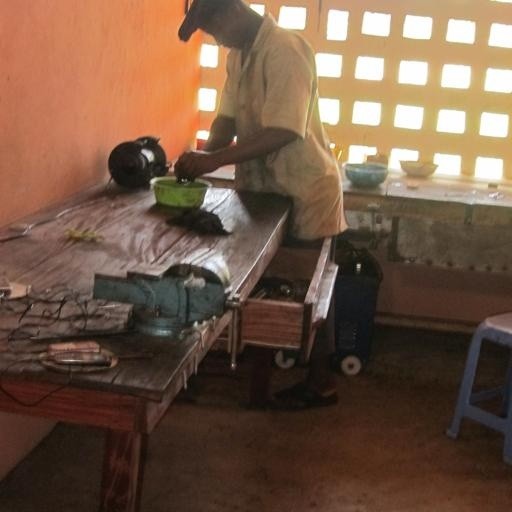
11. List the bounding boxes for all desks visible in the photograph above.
[1,176,341,510]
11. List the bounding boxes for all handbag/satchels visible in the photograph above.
[92,271,226,324]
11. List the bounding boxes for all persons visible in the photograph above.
[172,1,350,408]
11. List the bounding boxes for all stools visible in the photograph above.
[444,313,512,469]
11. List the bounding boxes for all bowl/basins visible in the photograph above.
[149,175,212,211]
[345,162,389,188]
[400,160,439,176]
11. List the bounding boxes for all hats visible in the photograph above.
[178,0,241,41]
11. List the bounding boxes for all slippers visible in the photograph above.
[267,382,338,411]
